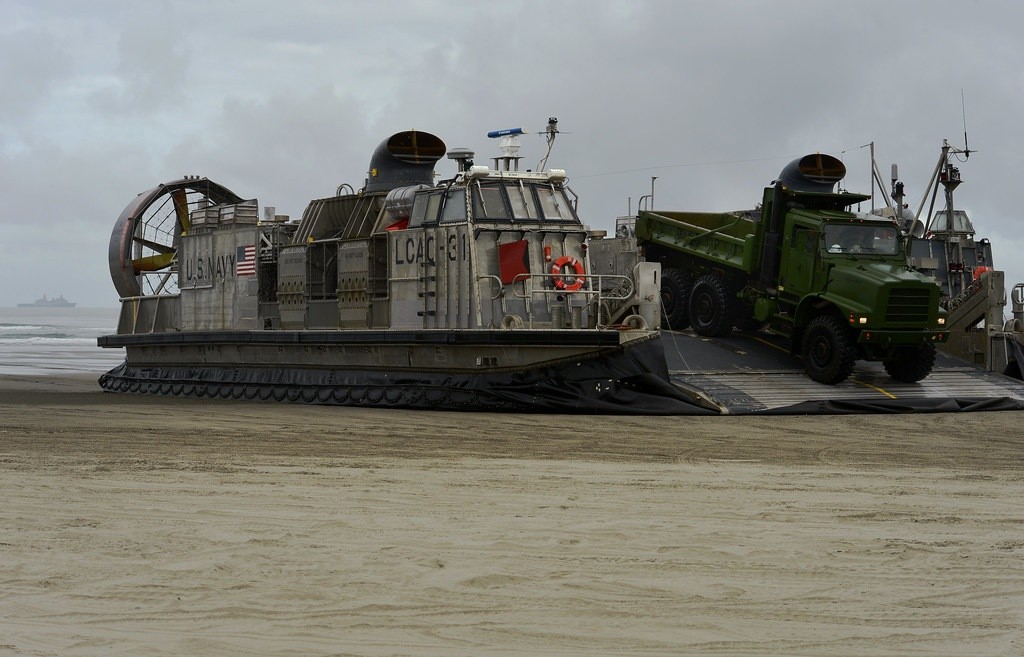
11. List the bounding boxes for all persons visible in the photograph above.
[849,230,867,253]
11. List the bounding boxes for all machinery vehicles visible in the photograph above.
[95,118,1024,413]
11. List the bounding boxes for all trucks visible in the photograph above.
[634,180,952,384]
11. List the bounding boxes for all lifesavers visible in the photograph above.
[974,266,993,280]
[551,256,585,291]
